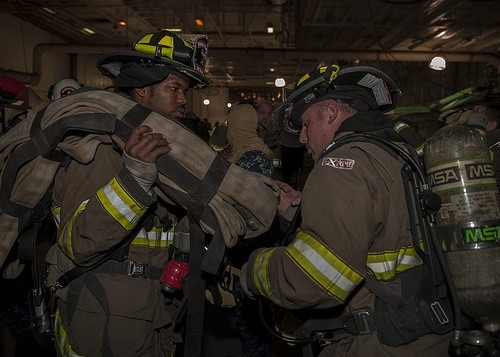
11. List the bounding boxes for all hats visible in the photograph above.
[271,59,400,148]
[96,29,220,98]
[2,76,33,112]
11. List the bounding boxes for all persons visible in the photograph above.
[25,31,213,356]
[240,57,457,357]
[182,102,500,357]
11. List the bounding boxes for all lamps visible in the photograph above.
[428,47,448,72]
